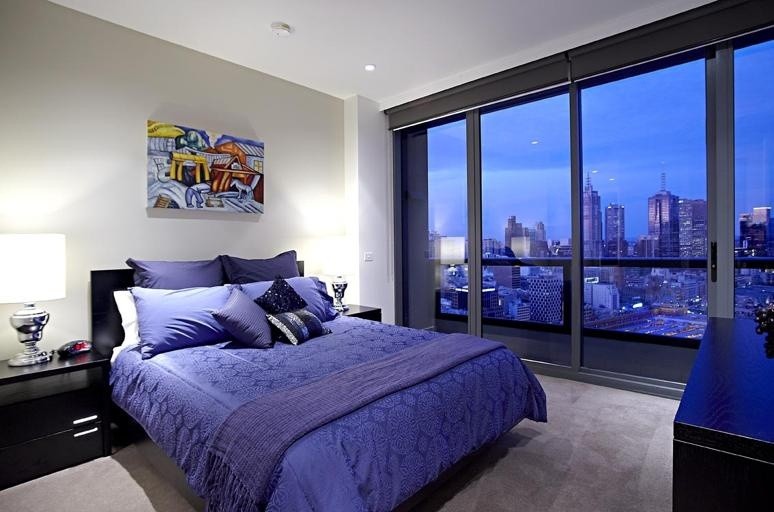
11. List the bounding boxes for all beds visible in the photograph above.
[88,260,547,512]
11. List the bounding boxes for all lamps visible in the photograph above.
[0,233,66,368]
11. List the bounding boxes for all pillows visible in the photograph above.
[111,250,340,359]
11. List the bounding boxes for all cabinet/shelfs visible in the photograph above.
[673,316,774,511]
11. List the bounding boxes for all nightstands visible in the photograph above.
[0,346,112,491]
